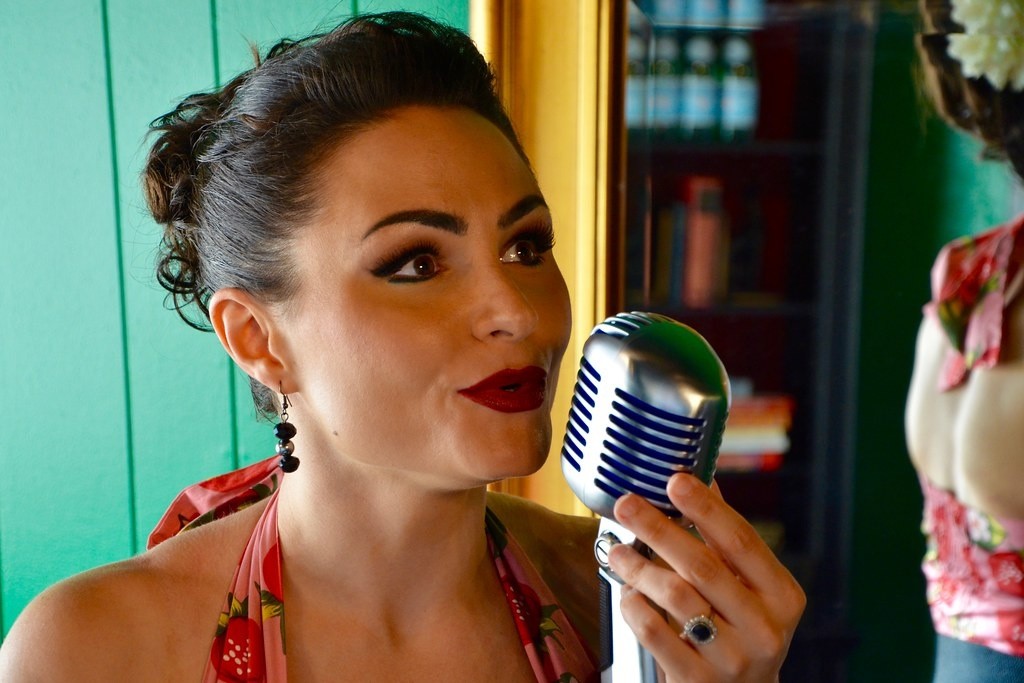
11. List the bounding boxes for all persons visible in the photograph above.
[906,0,1022,683]
[0,12,806,683]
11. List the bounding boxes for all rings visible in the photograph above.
[678,610,715,643]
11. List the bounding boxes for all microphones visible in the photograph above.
[561,311,732,568]
